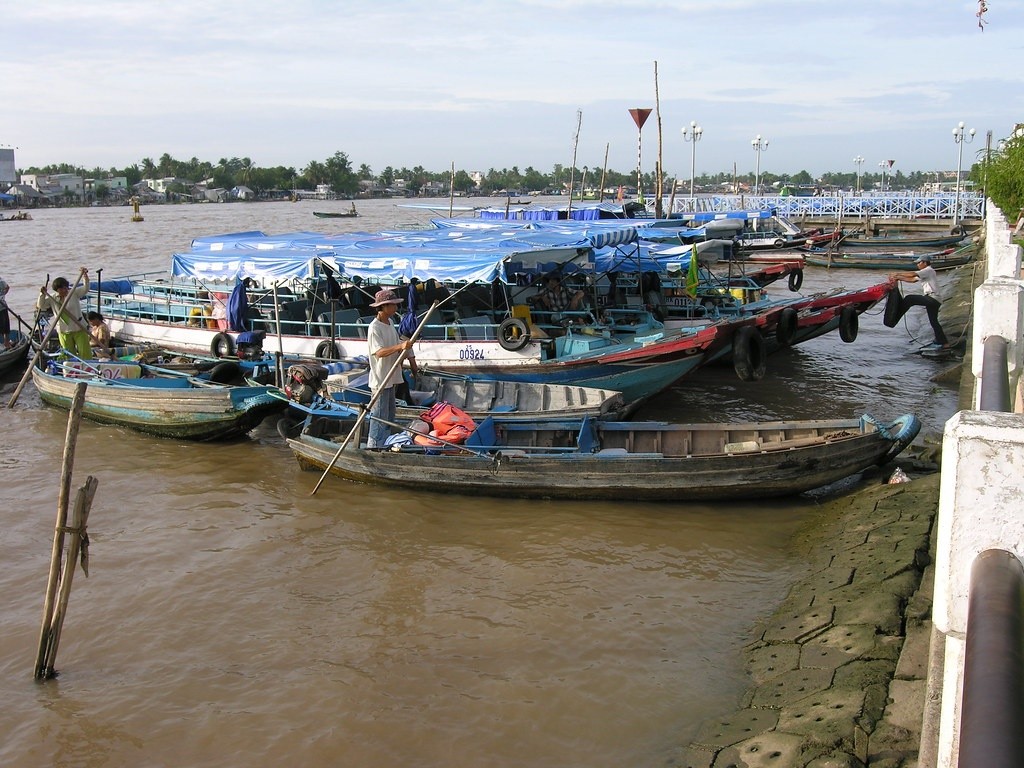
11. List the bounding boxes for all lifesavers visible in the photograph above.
[777,308,801,346]
[497,318,530,351]
[774,239,784,249]
[882,412,922,464]
[839,305,858,344]
[732,324,768,381]
[787,268,804,292]
[188,305,218,329]
[951,226,963,237]
[210,332,233,357]
[884,290,903,327]
[315,340,339,365]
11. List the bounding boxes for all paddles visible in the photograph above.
[1,268,119,409]
[312,299,439,496]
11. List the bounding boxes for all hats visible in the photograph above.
[913,254,932,264]
[368,290,405,308]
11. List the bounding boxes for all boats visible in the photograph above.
[0,326,31,369]
[310,207,358,218]
[281,414,923,506]
[17,59,923,442]
[131,201,146,224]
[504,199,532,206]
[0,212,32,220]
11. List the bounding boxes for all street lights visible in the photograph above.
[852,154,866,198]
[877,160,889,191]
[749,134,773,199]
[679,120,711,201]
[950,122,976,227]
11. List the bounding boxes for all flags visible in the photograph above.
[686,245,698,300]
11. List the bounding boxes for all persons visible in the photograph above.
[87,312,109,348]
[0,213,6,220]
[38,267,93,360]
[527,275,584,321]
[395,350,418,404]
[894,256,948,344]
[0,276,11,348]
[16,211,22,219]
[368,291,413,450]
